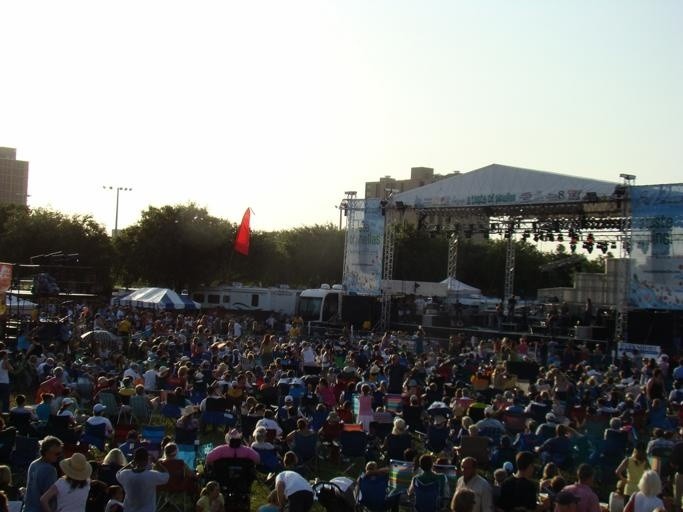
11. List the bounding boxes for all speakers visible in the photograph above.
[589,325,609,340]
[448,318,471,328]
[602,317,615,340]
[422,314,440,327]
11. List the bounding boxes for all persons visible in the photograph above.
[2,290,683,511]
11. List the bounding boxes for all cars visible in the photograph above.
[320,283,330,289]
[5,295,38,318]
[332,283,343,290]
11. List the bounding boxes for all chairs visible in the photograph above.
[0,306,681,511]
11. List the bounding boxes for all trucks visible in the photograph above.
[297,289,398,331]
[189,289,301,315]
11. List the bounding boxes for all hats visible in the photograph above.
[63,398,74,404]
[58,453,93,480]
[94,404,107,412]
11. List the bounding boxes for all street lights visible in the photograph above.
[103,185,133,234]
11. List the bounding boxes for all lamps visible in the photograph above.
[378,199,634,255]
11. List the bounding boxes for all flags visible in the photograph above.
[233,208,252,256]
